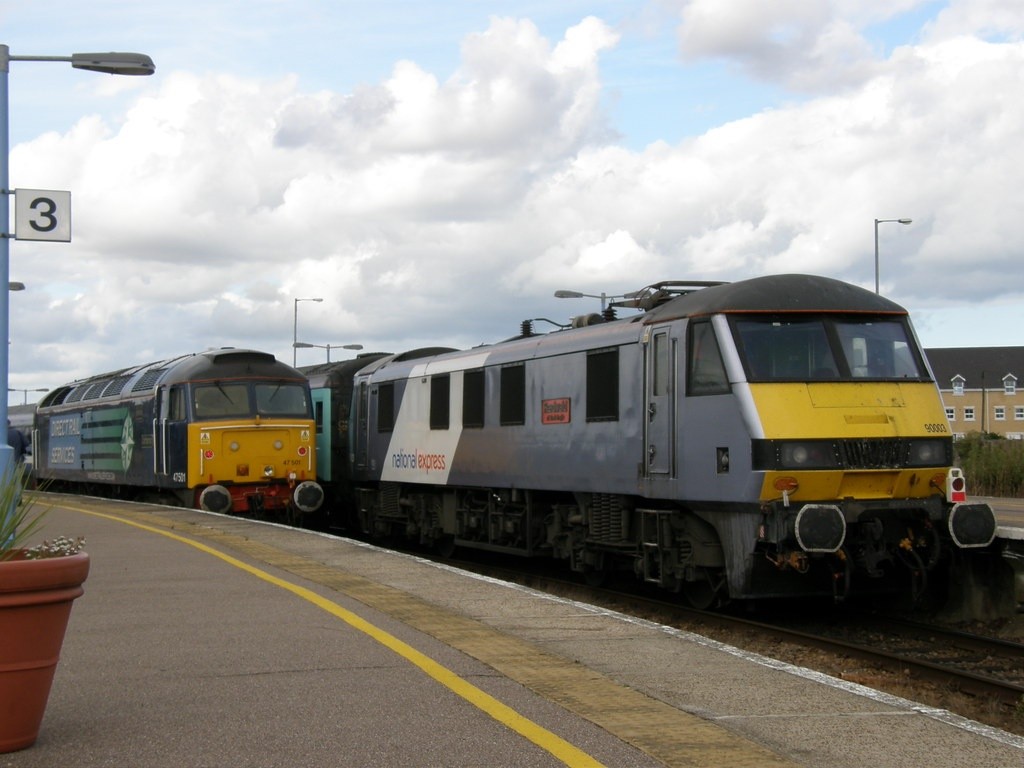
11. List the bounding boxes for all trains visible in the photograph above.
[300,272,968,615]
[32,345,324,520]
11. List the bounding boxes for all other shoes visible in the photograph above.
[16,497,22,506]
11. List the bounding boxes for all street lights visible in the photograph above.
[293,297,324,368]
[7,388,51,405]
[553,289,651,320]
[874,217,913,295]
[1,46,157,551]
[292,342,363,365]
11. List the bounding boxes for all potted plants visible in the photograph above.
[0,445,89,757]
